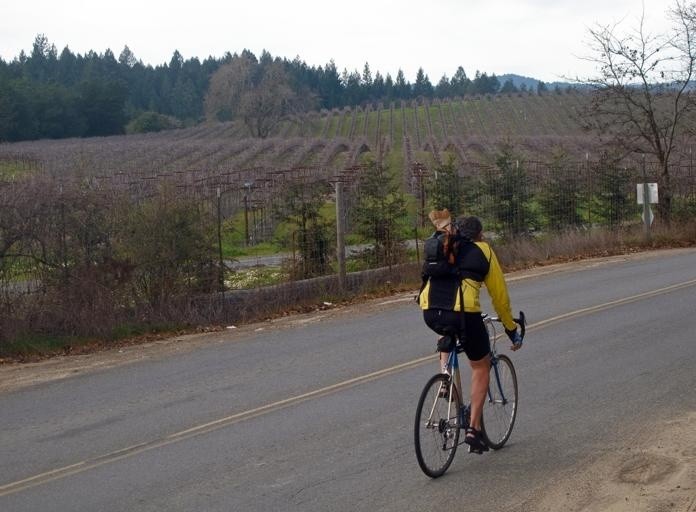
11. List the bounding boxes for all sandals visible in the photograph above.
[464,425,489,452]
[438,384,454,403]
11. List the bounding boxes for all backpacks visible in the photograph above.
[422,221,471,280]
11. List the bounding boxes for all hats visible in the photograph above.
[463,216,481,239]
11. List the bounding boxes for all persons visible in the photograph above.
[420,215,522,451]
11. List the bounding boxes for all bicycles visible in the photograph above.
[414,311,527,478]
